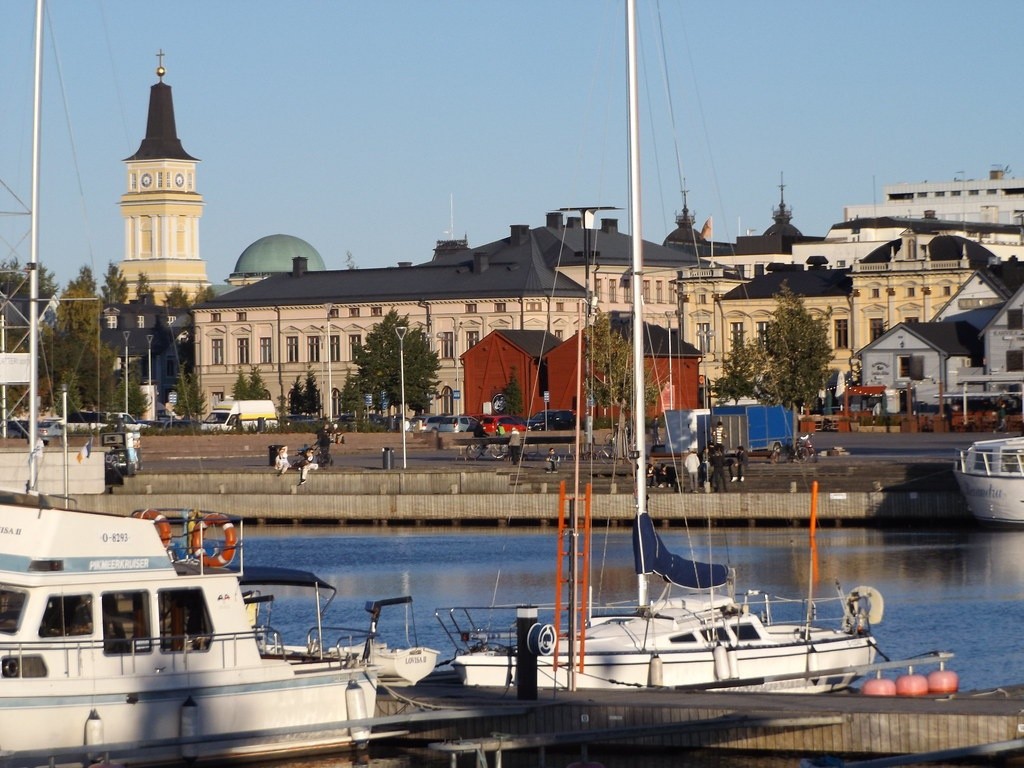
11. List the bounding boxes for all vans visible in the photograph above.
[200,400,278,431]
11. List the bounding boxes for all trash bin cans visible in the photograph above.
[258,417,266,431]
[383,447,394,469]
[389,416,396,429]
[269,445,283,466]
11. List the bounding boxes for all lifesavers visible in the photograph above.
[191,513,237,567]
[845,585,884,624]
[133,510,172,550]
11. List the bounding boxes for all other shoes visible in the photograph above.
[691,490,698,493]
[740,476,744,481]
[668,484,671,488]
[731,477,737,482]
[658,484,663,488]
[300,479,306,485]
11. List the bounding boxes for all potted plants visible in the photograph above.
[889,419,900,432]
[873,414,887,432]
[901,418,911,433]
[800,417,815,433]
[836,417,850,432]
[859,416,872,433]
[849,419,859,432]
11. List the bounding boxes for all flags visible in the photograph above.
[77,440,92,463]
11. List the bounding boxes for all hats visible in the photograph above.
[690,448,695,452]
[737,446,743,450]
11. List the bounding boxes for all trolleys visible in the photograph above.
[292,439,320,466]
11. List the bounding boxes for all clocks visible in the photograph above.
[175,174,184,187]
[141,174,152,188]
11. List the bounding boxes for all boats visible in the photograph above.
[265,643,441,686]
[952,437,1024,525]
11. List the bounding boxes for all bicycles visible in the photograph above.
[466,443,506,459]
[564,433,615,464]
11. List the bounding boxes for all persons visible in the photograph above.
[474,419,522,464]
[274,423,344,484]
[645,415,750,493]
[941,404,954,432]
[546,448,558,473]
[994,403,1008,434]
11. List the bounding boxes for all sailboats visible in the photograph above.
[432,0,877,692]
[0,0,382,767]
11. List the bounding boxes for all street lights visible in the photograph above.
[323,303,332,422]
[665,311,674,410]
[395,327,407,468]
[146,335,154,420]
[123,331,130,414]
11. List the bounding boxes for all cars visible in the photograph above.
[139,420,200,429]
[0,419,30,438]
[481,416,528,432]
[36,421,65,437]
[525,410,577,431]
[282,414,489,433]
[57,411,151,431]
